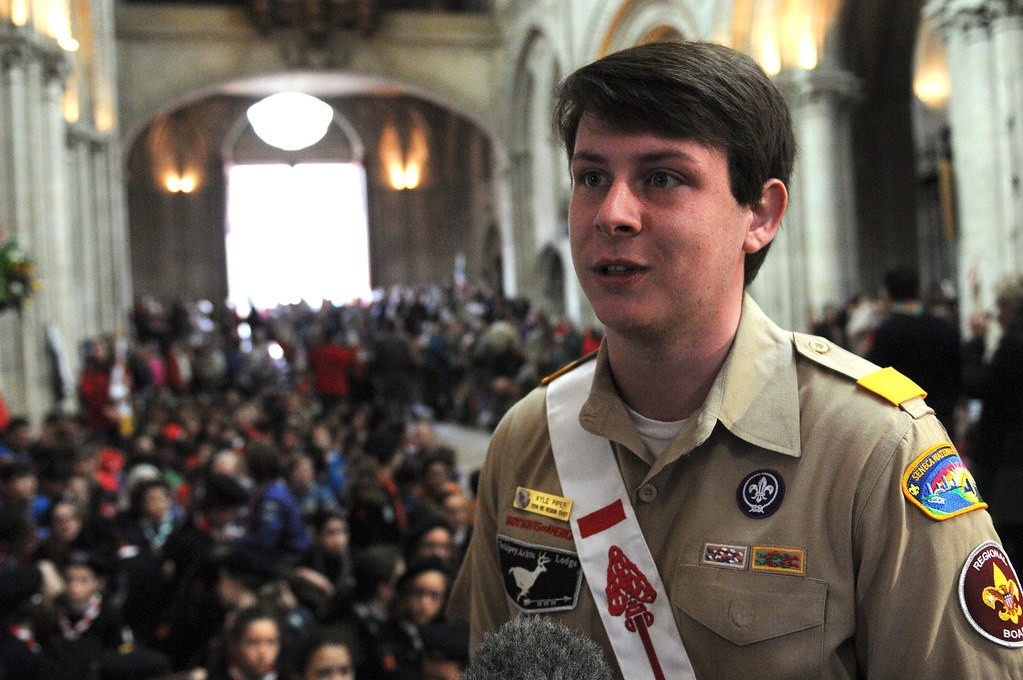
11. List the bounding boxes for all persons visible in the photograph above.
[0,282,604,680]
[444,42,1023,680]
[816,275,1023,583]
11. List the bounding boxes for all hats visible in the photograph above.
[395,556,448,591]
[64,550,104,576]
